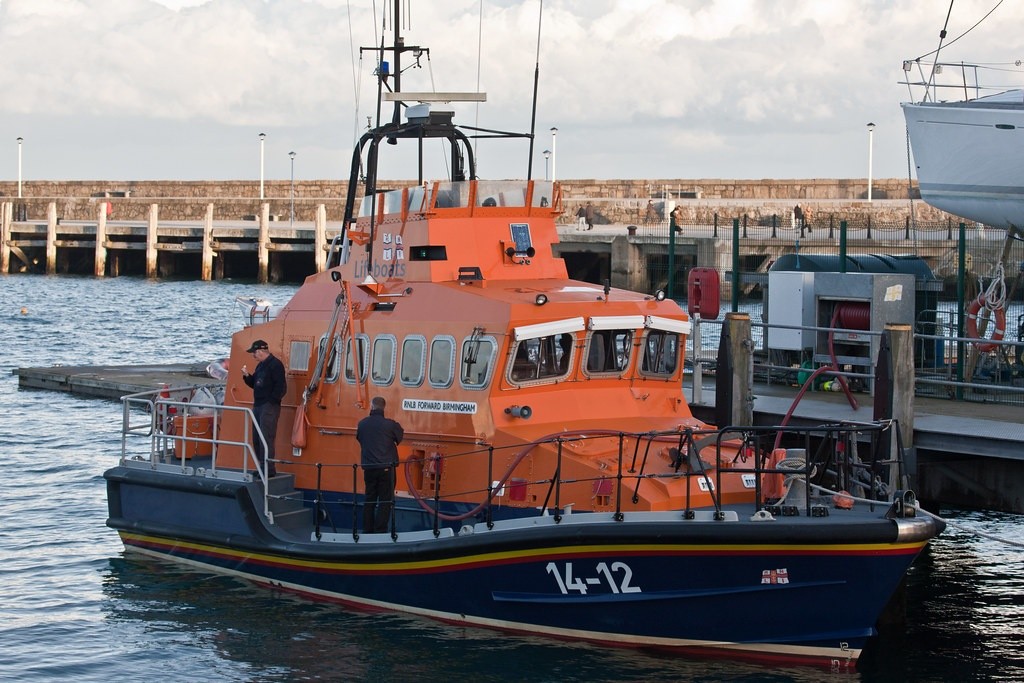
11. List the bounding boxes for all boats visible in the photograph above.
[103,0,949,683]
[899,0,1024,234]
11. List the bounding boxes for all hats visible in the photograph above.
[246,339,268,353]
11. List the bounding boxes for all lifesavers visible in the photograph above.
[966,295,1007,354]
[156,385,177,435]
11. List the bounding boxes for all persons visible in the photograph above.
[241,340,287,478]
[356,397,403,534]
[801,207,814,233]
[794,203,802,234]
[575,204,586,231]
[669,206,685,236]
[585,202,594,230]
[644,200,656,225]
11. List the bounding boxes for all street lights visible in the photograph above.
[542,149,551,180]
[16,137,25,198]
[288,150,296,227]
[257,132,266,198]
[550,126,559,182]
[866,122,876,203]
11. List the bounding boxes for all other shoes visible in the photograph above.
[678,232,685,236]
[582,229,585,231]
[576,228,580,231]
[257,462,276,478]
